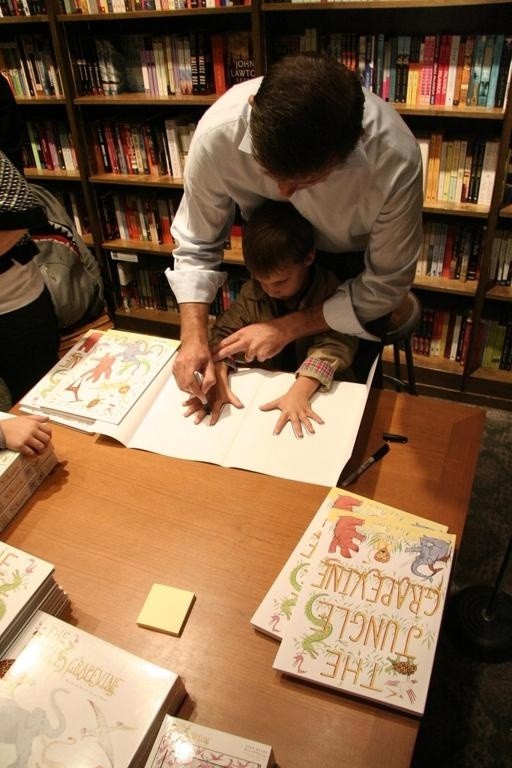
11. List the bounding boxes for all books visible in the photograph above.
[39,179,87,237]
[97,187,188,245]
[86,109,202,179]
[60,19,251,94]
[418,219,486,284]
[0,0,249,11]
[408,287,471,371]
[109,246,250,318]
[18,326,184,436]
[487,224,510,288]
[415,128,501,212]
[0,25,62,97]
[0,410,60,539]
[473,313,508,371]
[266,20,512,110]
[6,106,77,171]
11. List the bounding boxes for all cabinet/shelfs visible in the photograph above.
[6,29,64,99]
[14,115,76,168]
[267,14,509,112]
[104,190,251,253]
[413,126,493,208]
[113,262,251,327]
[82,116,219,182]
[415,218,481,282]
[494,229,512,289]
[478,307,512,381]
[60,16,258,101]
[411,294,471,365]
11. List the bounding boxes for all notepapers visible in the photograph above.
[136,582,195,638]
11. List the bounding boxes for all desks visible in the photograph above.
[2,330,487,768]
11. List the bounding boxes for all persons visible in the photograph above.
[1,76,61,405]
[165,50,423,403]
[0,410,53,456]
[178,201,363,438]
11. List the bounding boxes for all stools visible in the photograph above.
[379,283,428,396]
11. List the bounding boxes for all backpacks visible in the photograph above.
[28,183,105,334]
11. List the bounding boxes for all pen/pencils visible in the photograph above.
[193,370,212,416]
[342,443,390,488]
